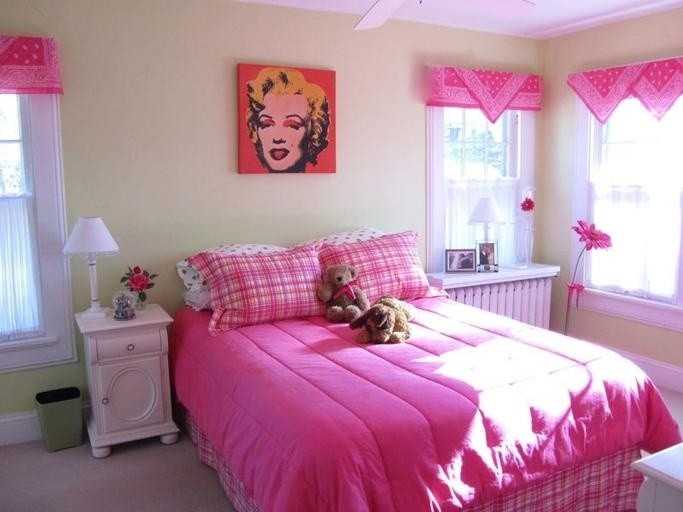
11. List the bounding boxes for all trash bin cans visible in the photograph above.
[33,386,83,453]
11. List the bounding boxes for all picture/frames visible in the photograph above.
[476,241,499,273]
[445,249,477,273]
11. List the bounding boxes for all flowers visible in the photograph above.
[562,222,614,335]
[120,266,158,301]
[520,187,538,215]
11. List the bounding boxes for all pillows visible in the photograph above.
[291,228,393,248]
[331,229,432,304]
[177,245,288,309]
[187,245,326,335]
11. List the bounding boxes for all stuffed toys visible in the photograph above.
[316,263,369,324]
[347,294,418,345]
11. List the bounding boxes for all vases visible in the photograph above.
[133,292,146,310]
[514,216,537,270]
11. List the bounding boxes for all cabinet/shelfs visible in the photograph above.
[75,306,181,459]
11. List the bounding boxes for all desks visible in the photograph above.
[628,443,683,512]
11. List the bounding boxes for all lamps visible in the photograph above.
[62,216,119,321]
[468,198,503,243]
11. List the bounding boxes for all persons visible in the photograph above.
[242,66,332,174]
[479,243,493,264]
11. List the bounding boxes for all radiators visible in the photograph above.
[444,277,557,329]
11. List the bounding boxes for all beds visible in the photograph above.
[168,227,683,512]
